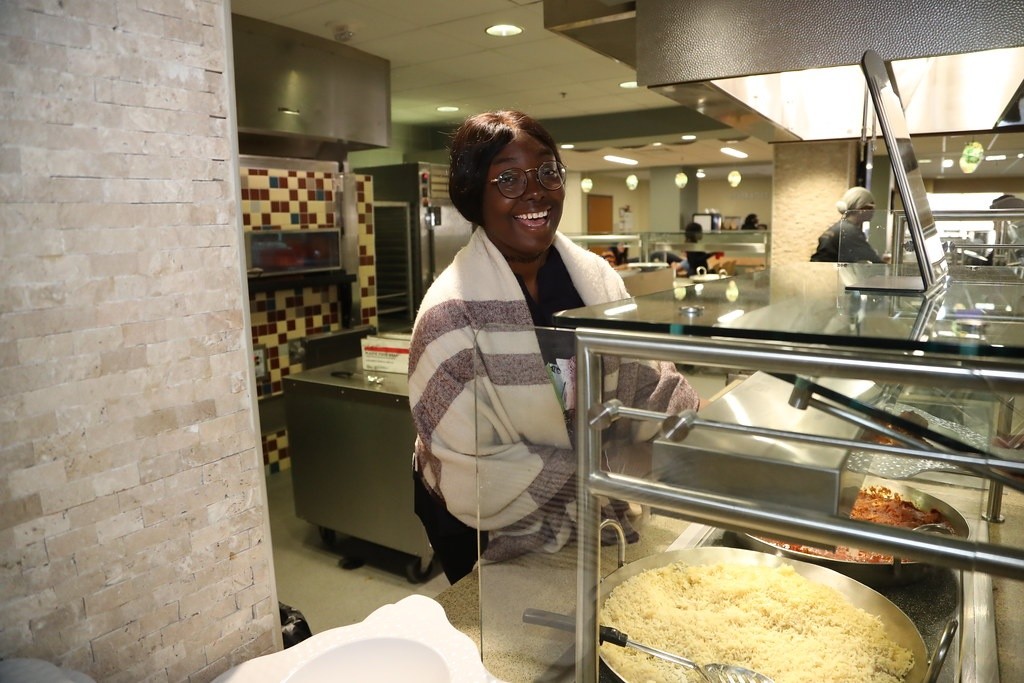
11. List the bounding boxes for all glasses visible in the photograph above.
[865,201,876,209]
[485,160,568,199]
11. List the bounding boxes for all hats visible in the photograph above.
[837,187,873,214]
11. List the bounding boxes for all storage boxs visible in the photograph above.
[359,333,411,374]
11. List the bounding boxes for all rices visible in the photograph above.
[598,562,915,683]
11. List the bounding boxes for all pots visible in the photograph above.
[689,266,732,285]
[595,517,958,683]
[736,469,967,579]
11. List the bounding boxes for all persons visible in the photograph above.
[685,223,725,277]
[407,108,699,586]
[971,194,1024,358]
[741,214,758,230]
[809,187,884,264]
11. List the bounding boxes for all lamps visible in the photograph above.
[963,135,984,162]
[728,165,741,187]
[580,169,592,192]
[958,156,977,172]
[674,166,687,187]
[625,170,637,190]
